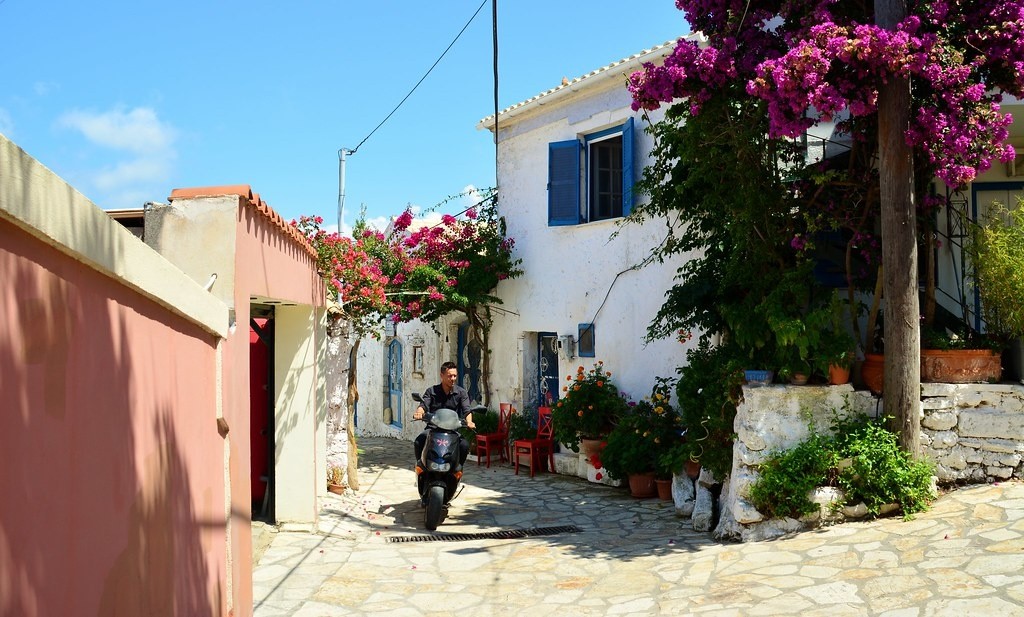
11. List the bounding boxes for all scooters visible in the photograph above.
[411,392,487,531]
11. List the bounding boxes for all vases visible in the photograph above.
[583,436,604,461]
[625,457,702,504]
[327,481,348,494]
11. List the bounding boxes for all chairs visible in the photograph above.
[477,402,512,468]
[514,407,557,476]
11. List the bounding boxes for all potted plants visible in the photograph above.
[457,408,499,456]
[748,416,939,526]
[745,289,1003,396]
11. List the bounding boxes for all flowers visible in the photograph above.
[553,351,614,415]
[324,460,349,479]
[620,386,685,446]
[581,434,612,482]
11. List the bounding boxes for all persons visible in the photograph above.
[412,362,476,482]
